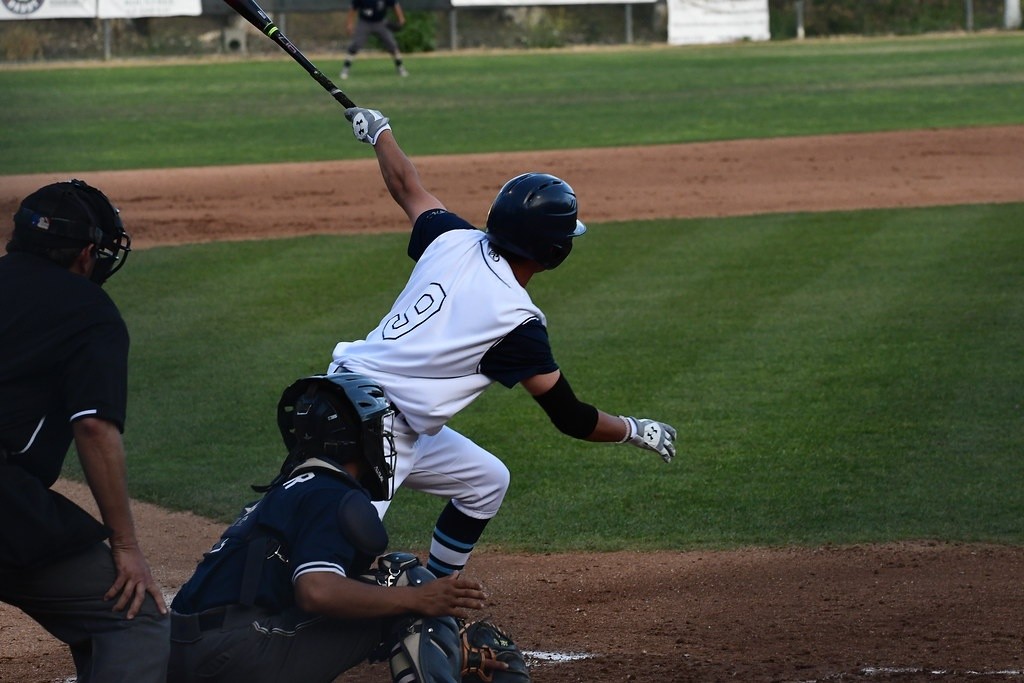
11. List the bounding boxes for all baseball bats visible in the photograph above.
[221,0,358,112]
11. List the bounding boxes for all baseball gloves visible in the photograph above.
[386,19,407,31]
[456,617,530,683]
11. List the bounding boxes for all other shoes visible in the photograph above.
[399,68,409,78]
[339,70,347,80]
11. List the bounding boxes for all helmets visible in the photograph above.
[277,372,398,502]
[15,179,132,286]
[486,172,586,271]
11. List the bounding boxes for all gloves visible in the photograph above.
[614,413,677,463]
[343,106,392,145]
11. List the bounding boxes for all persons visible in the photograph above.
[167,374,509,683]
[325,107,678,584]
[340,0,409,80]
[0,179,171,683]
